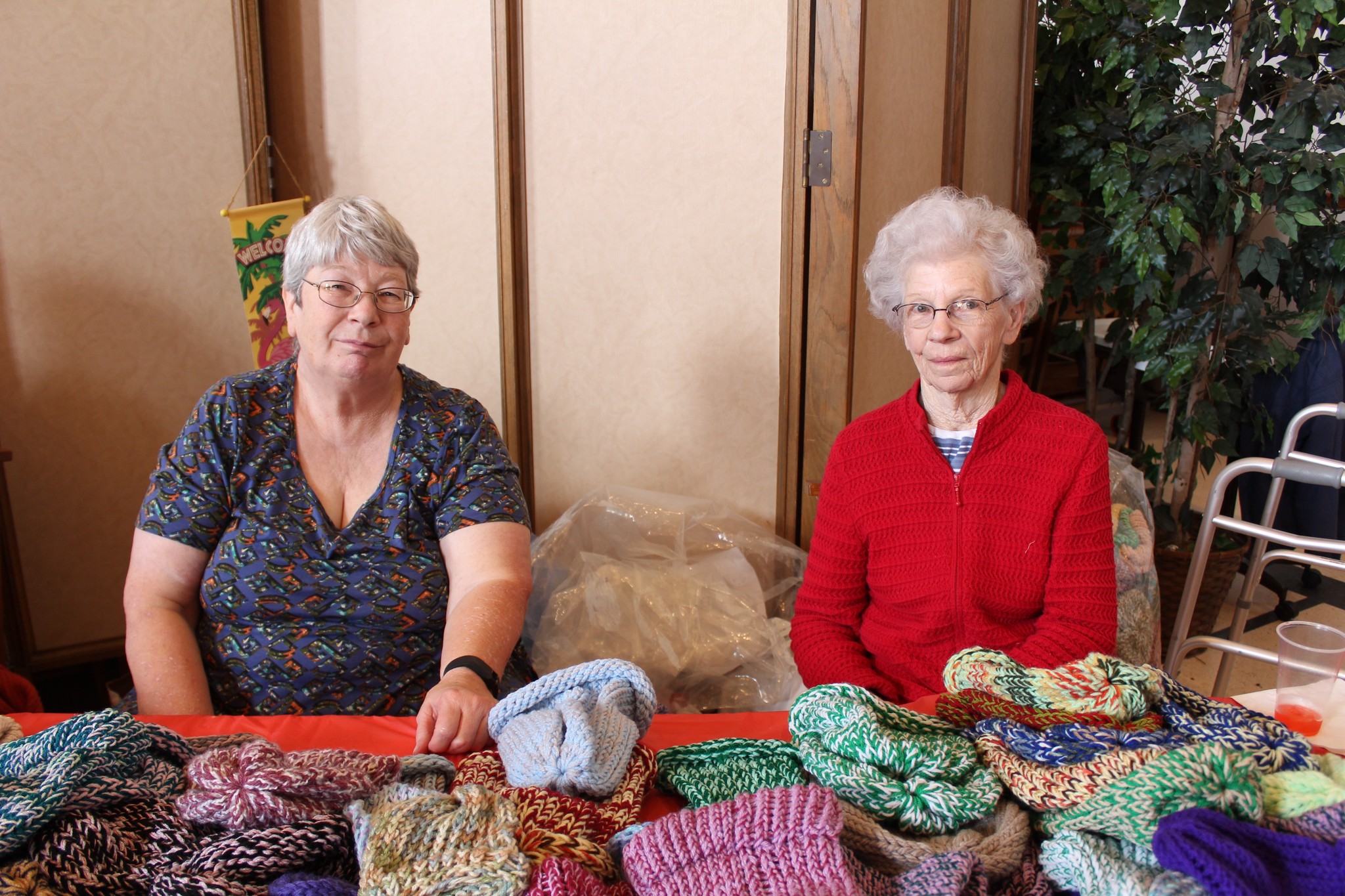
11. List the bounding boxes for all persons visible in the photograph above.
[784,181,1122,721]
[113,191,540,765]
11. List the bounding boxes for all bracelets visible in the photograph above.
[441,654,501,701]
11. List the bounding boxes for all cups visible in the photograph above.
[1274,620,1345,738]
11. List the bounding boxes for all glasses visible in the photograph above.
[893,292,1014,329]
[299,278,418,313]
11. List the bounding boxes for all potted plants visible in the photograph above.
[1027,0,1345,660]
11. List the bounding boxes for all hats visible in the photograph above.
[2,642,1345,896]
